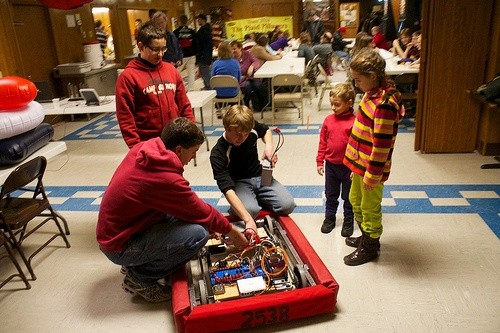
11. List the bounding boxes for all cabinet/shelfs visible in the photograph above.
[61,69,117,120]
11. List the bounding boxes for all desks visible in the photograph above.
[38,91,217,166]
[254,51,305,119]
[0,141,71,235]
[378,48,419,99]
[344,38,354,48]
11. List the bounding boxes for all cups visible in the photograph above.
[52,98,60,111]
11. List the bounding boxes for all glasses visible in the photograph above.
[144,41,167,53]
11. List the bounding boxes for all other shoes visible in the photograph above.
[345,235,363,247]
[121,273,172,303]
[321,217,336,234]
[120,265,129,275]
[344,246,376,266]
[341,217,354,237]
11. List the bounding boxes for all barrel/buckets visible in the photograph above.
[82,39,103,69]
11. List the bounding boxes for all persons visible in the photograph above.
[210,42,241,119]
[231,40,267,111]
[343,47,406,267]
[94,117,248,303]
[211,104,296,245]
[297,31,317,71]
[331,27,350,71]
[316,83,360,236]
[195,13,212,90]
[240,34,257,53]
[250,35,282,84]
[313,32,333,67]
[135,19,143,41]
[152,11,184,68]
[93,19,108,51]
[308,9,323,46]
[115,24,195,151]
[346,25,422,121]
[267,26,293,50]
[174,15,197,92]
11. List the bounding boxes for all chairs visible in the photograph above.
[271,52,360,125]
[210,75,245,127]
[0,155,72,280]
[0,230,32,289]
